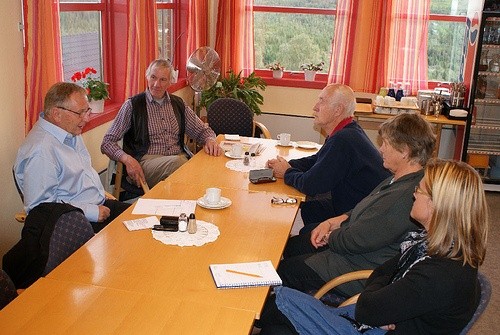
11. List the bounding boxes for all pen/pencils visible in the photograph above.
[226,270,263,278]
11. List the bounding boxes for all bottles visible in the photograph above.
[475,75,487,99]
[187,213,197,234]
[178,213,188,233]
[250,153,256,166]
[483,26,500,43]
[244,152,250,165]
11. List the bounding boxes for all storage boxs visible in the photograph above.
[354,98,372,116]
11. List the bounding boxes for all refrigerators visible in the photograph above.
[459,0,500,193]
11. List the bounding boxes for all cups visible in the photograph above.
[230,144,244,157]
[276,132,292,145]
[204,188,221,204]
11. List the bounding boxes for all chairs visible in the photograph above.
[313,269,492,335]
[193,98,271,156]
[21,202,94,277]
[113,133,189,201]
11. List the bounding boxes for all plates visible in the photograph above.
[224,152,249,159]
[276,140,293,146]
[296,141,319,150]
[197,196,232,210]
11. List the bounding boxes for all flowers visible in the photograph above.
[266,62,285,71]
[300,62,324,72]
[71,67,111,102]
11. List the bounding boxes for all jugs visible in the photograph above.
[479,49,489,71]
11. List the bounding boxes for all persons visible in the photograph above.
[99,58,223,189]
[258,157,488,335]
[263,84,392,224]
[275,113,435,297]
[13,82,133,235]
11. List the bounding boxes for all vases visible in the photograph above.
[89,97,104,113]
[304,70,317,81]
[171,70,179,83]
[273,70,282,79]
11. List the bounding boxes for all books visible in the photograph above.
[209,260,283,288]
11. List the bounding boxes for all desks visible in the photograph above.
[200,105,208,123]
[46,180,301,320]
[165,134,324,203]
[354,110,466,159]
[0,277,255,335]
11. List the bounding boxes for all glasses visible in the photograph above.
[414,185,430,197]
[56,106,93,118]
[271,196,297,205]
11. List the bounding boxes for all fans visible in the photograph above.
[186,46,221,154]
